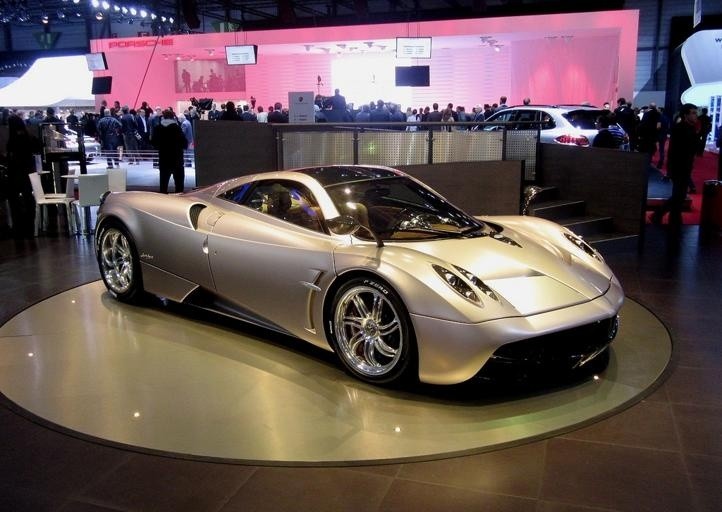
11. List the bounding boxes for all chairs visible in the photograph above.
[28,166,127,236]
[308,201,371,241]
[266,182,292,220]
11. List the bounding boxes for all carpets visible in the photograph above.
[0,279,681,468]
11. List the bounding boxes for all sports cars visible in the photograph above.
[94,164,625,387]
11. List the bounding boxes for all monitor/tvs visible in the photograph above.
[396,37,432,58]
[86,52,108,71]
[395,65,429,87]
[225,45,257,65]
[92,76,112,94]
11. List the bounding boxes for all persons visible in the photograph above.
[181,67,245,92]
[0,96,65,237]
[323,88,347,109]
[154,110,189,194]
[580,98,717,239]
[64,98,530,169]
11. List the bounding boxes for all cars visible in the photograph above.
[470,104,631,153]
[64,128,102,165]
